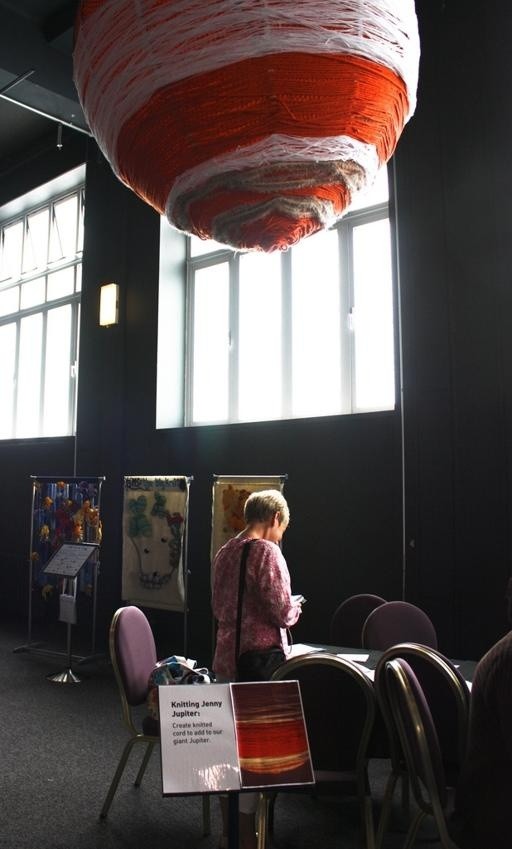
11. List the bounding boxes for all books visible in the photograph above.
[290,595,307,606]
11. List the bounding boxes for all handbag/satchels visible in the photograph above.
[238,646,286,681]
[144,654,213,722]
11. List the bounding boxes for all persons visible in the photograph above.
[211,488,303,848]
